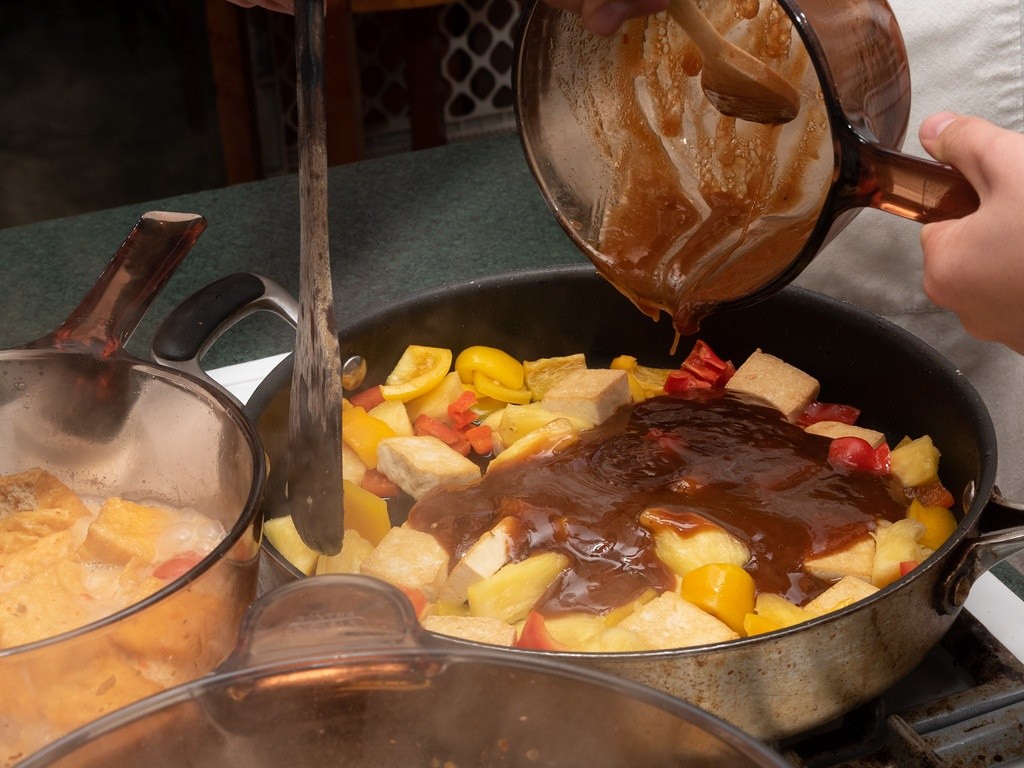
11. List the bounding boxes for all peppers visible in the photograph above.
[336,344,961,651]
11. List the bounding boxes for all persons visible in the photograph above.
[546,0,1024,514]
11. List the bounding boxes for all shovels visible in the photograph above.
[286,0,345,558]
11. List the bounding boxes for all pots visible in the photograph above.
[510,0,980,326]
[0,208,270,768]
[11,574,797,768]
[142,265,1024,757]
